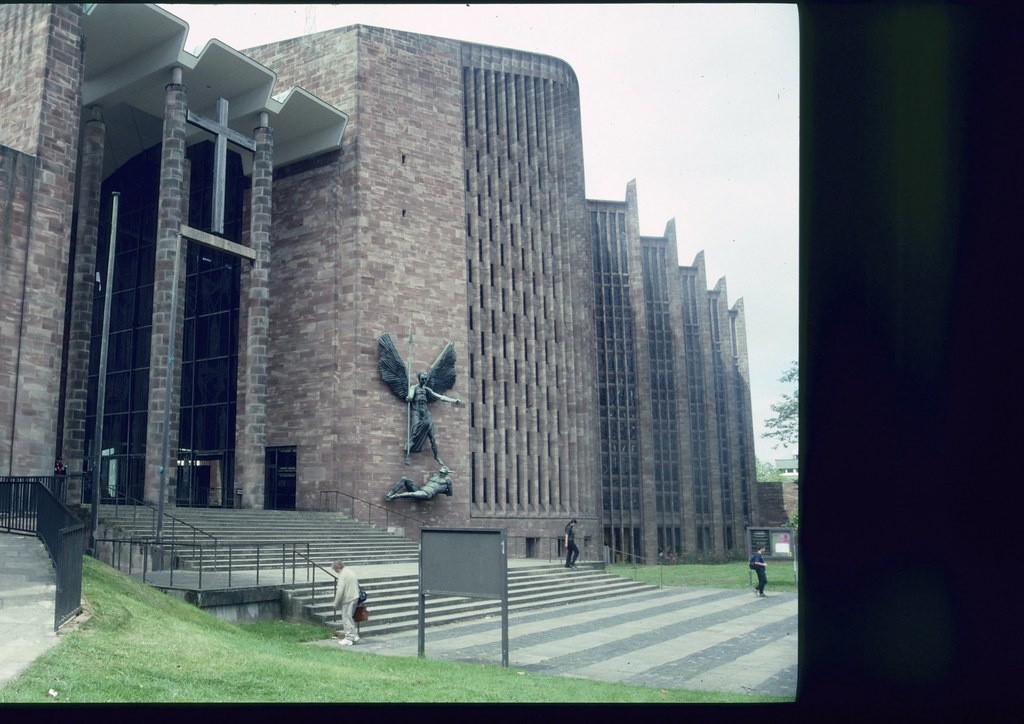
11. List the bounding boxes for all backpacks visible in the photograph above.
[749,554,756,568]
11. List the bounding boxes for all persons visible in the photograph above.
[384,465,453,502]
[55,455,67,475]
[331,559,361,646]
[753,544,768,597]
[405,369,461,466]
[564,519,579,567]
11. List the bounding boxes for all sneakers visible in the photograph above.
[354,636,360,643]
[339,639,353,646]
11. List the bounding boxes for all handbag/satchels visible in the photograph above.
[354,606,368,622]
[359,592,367,603]
